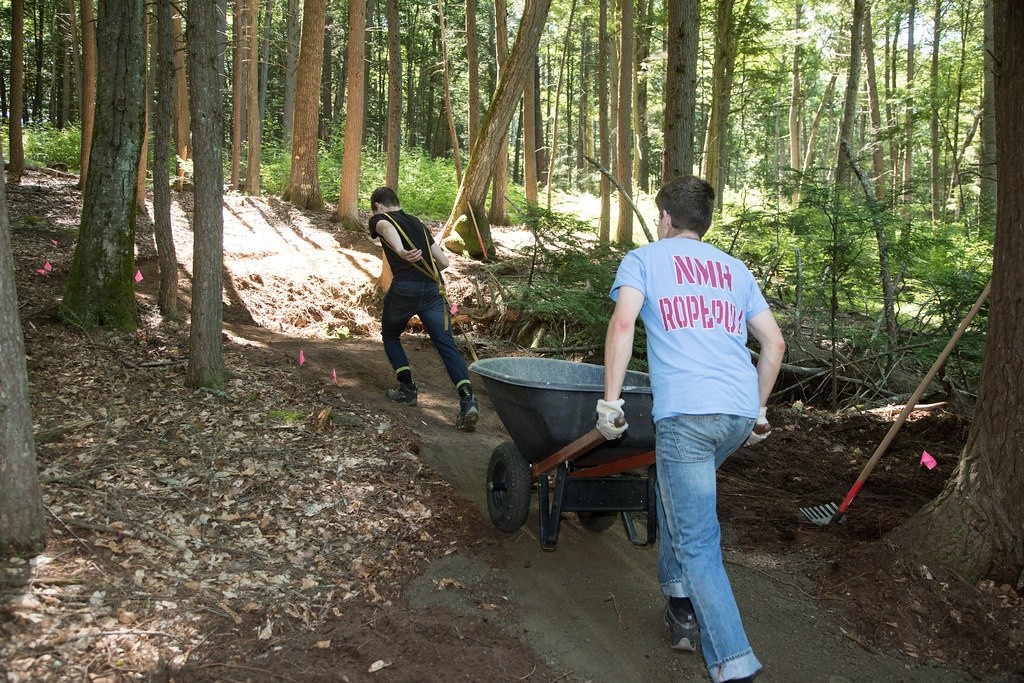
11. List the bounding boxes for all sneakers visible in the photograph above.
[387,383,418,406]
[663,600,699,653]
[455,393,480,432]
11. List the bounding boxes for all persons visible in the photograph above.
[369,187,479,432]
[596,175,785,683]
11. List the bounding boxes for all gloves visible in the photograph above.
[743,406,772,447]
[595,398,629,441]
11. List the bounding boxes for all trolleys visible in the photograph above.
[468,355,770,552]
